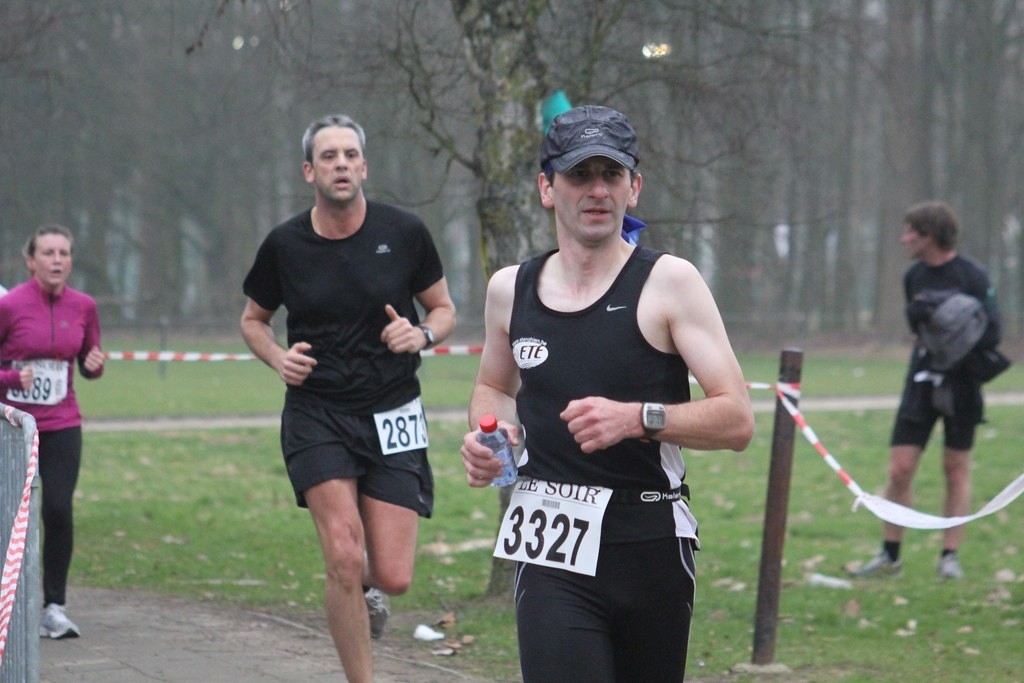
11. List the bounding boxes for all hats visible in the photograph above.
[540,104,640,175]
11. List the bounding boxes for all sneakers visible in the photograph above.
[38,603,81,639]
[361,551,391,639]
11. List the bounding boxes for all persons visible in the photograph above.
[0,223,107,639]
[460,105,755,683]
[240,112,457,683]
[844,204,1004,581]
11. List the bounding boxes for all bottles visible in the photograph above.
[476,415,518,487]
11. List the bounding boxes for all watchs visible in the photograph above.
[641,402,667,438]
[419,325,434,350]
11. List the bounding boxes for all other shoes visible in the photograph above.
[935,551,965,580]
[844,547,903,580]
